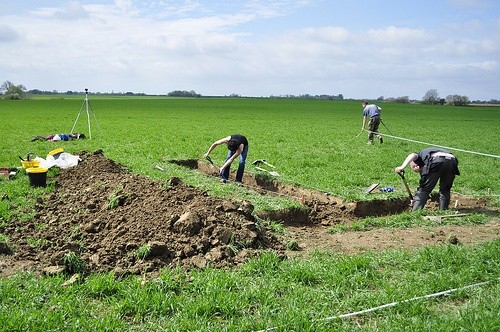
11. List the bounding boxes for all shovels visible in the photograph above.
[253,159,276,168]
[256,167,280,176]
[397,169,415,209]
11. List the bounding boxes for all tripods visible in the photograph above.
[70,94,102,140]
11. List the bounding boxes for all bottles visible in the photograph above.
[60,135,68,141]
[53,134,61,141]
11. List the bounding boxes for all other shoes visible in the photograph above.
[367,139,375,145]
[378,136,384,145]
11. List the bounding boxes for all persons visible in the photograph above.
[203,134,249,187]
[362,101,385,144]
[396,148,459,212]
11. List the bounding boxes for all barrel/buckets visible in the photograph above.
[49,148,65,160]
[26,168,48,188]
[21,159,40,168]
